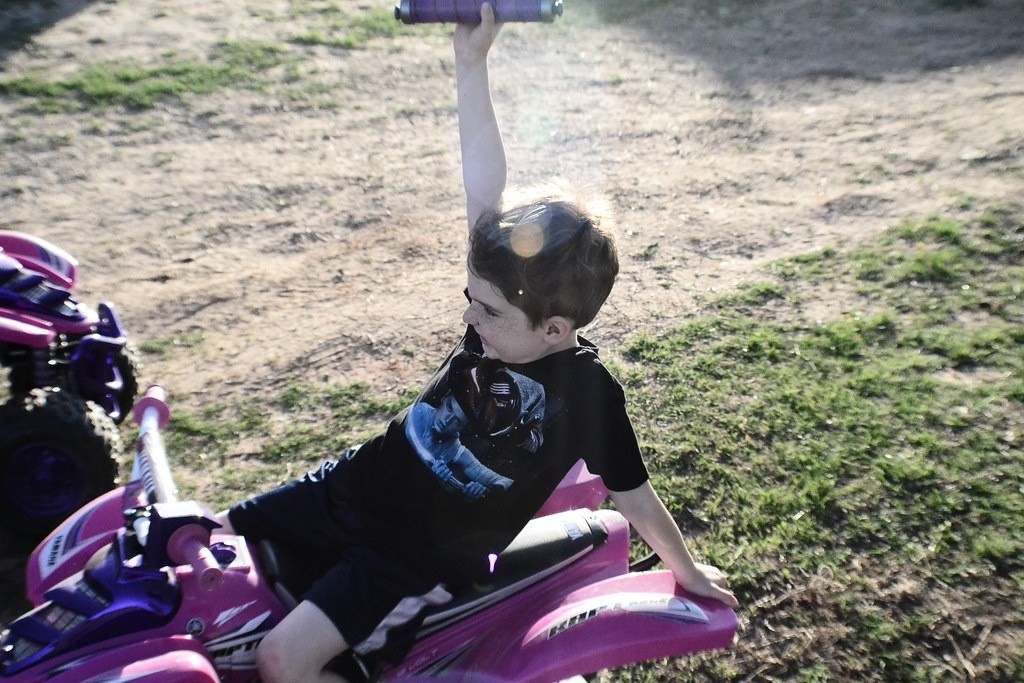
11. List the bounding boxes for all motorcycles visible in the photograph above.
[1,382,747,683]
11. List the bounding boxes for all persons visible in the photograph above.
[202,2,739,683]
